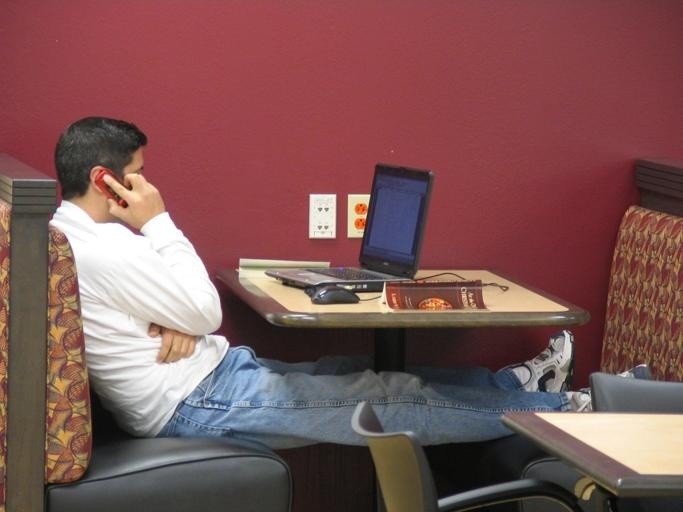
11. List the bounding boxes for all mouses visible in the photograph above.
[312,286,359,304]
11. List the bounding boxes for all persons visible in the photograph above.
[51,115,652,447]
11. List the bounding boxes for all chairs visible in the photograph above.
[588,369,683,418]
[351,399,586,511]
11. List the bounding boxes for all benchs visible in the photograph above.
[47,224,294,512]
[489,204,683,512]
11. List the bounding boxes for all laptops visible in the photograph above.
[265,163,434,292]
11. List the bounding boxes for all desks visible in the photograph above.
[214,264,590,375]
[502,411,683,512]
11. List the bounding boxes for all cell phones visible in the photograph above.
[95,170,131,208]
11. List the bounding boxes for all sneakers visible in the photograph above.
[570,361,654,413]
[509,327,578,394]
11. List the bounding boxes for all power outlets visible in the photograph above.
[349,194,371,238]
[308,193,337,239]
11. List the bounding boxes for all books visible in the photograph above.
[379,277,488,313]
